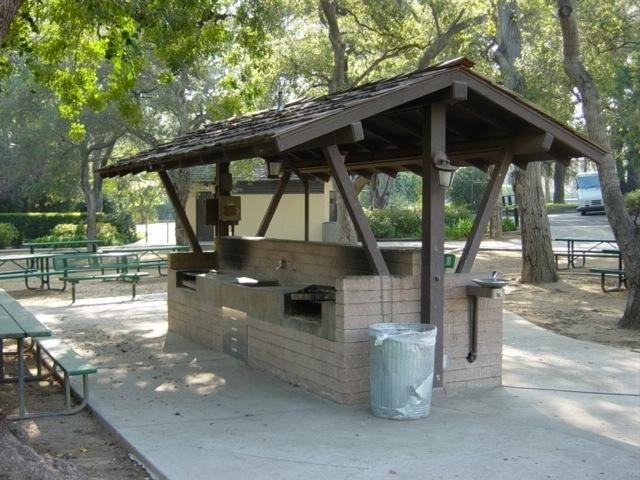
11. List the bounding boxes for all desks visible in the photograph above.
[0,286,53,419]
[551,233,623,270]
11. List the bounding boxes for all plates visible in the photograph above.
[471,278,512,289]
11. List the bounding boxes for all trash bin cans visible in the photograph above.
[369,323,437,419]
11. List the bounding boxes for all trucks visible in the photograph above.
[575,171,607,217]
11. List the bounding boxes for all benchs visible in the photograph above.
[586,266,627,291]
[0,238,192,303]
[28,334,96,415]
[553,251,625,272]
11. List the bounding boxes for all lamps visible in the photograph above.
[265,158,285,179]
[433,153,460,186]
